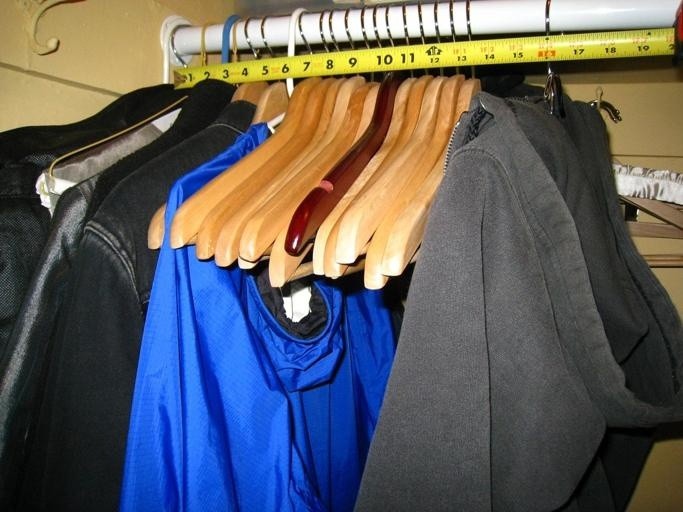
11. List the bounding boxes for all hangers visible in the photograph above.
[33,0,564,288]
